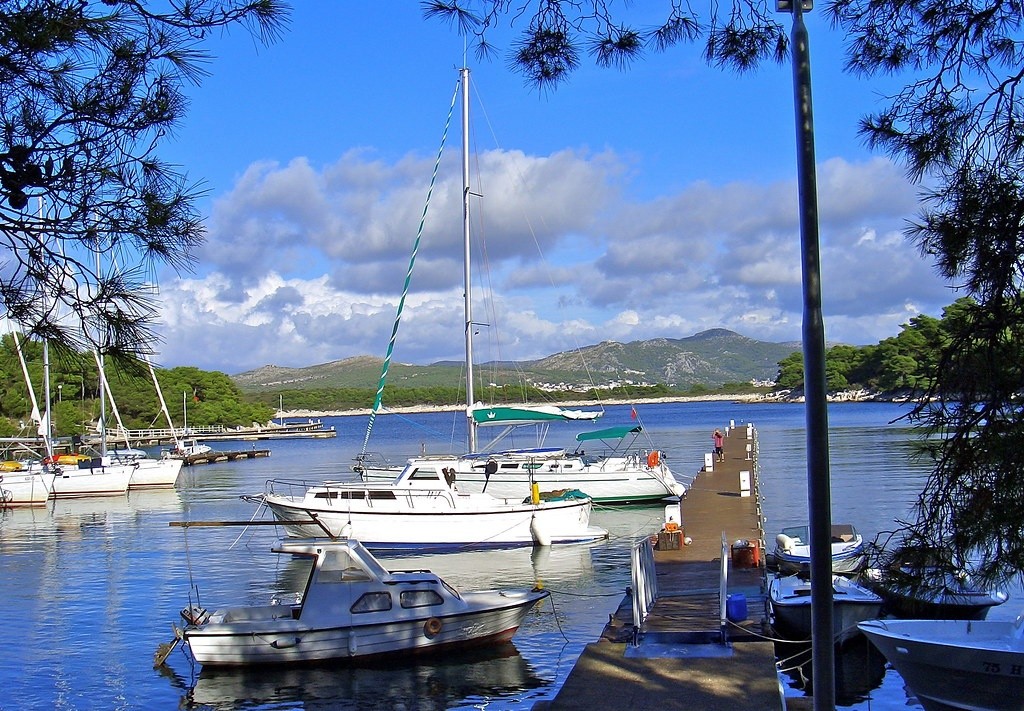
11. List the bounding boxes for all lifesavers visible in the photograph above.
[425,618,442,635]
[647,451,659,466]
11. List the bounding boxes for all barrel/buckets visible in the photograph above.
[726,593,748,622]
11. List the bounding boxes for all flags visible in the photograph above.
[632,409,637,419]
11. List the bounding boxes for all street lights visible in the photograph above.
[58,384,62,402]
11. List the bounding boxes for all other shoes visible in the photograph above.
[722,458,724,462]
[716,458,720,462]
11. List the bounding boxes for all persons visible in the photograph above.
[712,428,725,462]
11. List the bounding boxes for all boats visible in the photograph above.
[771,522,872,580]
[770,623,889,707]
[763,570,885,649]
[240,478,612,547]
[861,530,1010,621]
[160,390,212,463]
[857,611,1024,711]
[175,640,555,711]
[151,508,553,672]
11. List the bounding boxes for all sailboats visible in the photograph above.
[351,32,689,506]
[0,192,186,508]
